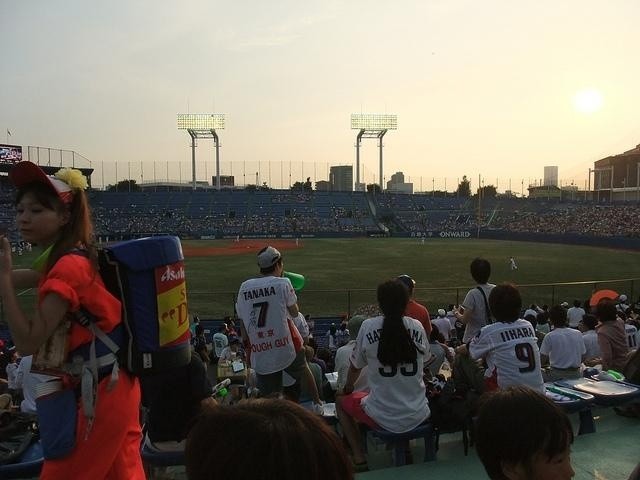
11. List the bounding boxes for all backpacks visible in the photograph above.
[76,235,191,374]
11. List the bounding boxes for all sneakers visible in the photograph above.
[350,461,369,472]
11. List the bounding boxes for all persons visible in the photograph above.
[473,385,575,480]
[1,162,147,479]
[1,245,638,470]
[1,177,639,256]
[185,400,353,479]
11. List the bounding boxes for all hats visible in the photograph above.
[8,162,76,205]
[561,301,568,306]
[438,309,446,316]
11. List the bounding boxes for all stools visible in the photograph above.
[1,190,640,253]
[1,320,640,480]
